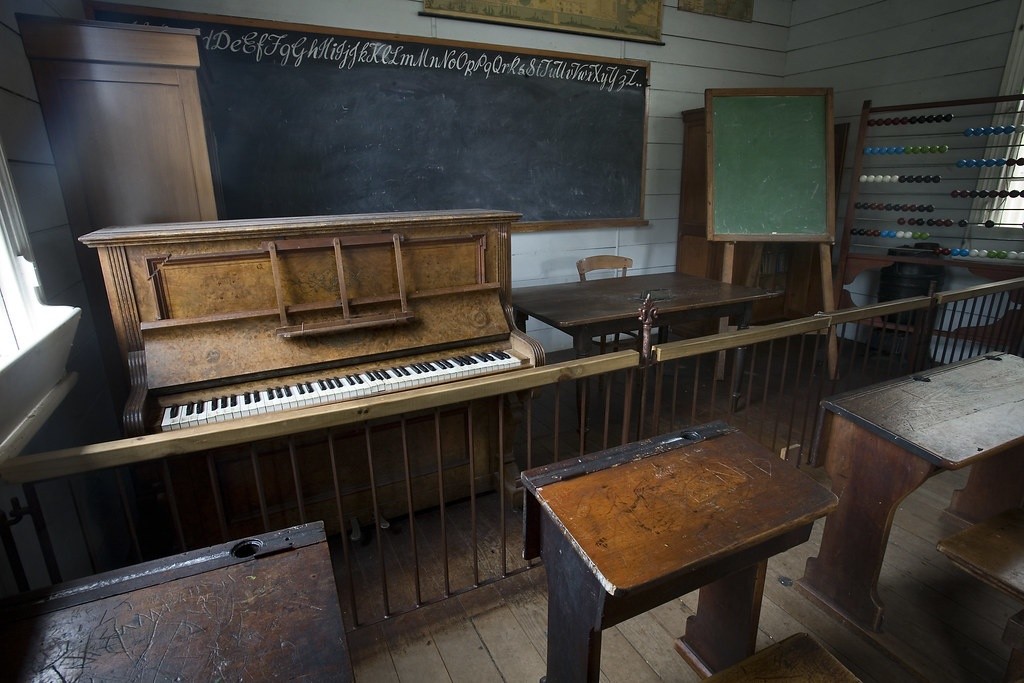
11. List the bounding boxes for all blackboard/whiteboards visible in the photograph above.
[703,86,837,243]
[84,1,649,231]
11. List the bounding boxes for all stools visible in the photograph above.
[701,633,865,682]
[935,506,1024,683]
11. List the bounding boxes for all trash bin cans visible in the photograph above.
[870,242,944,357]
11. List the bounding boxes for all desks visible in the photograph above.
[798,348,1024,636]
[11,520,363,683]
[521,421,841,683]
[514,273,786,448]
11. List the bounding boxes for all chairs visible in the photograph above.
[577,255,640,395]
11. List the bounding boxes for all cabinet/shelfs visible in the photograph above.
[671,108,790,337]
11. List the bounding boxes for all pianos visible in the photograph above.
[75,206,548,551]
